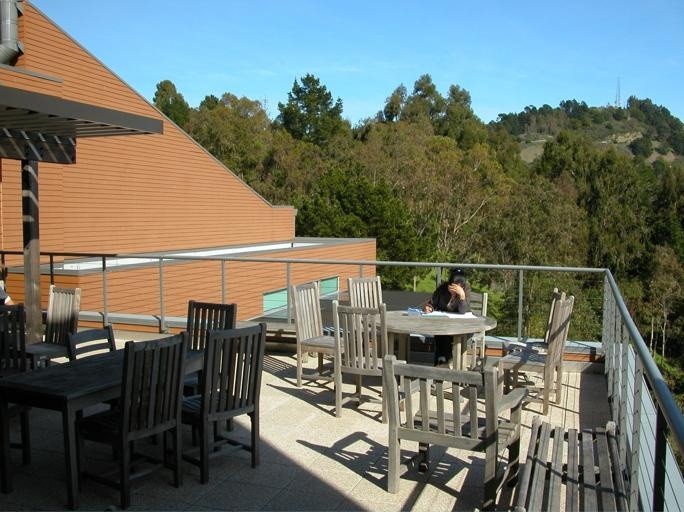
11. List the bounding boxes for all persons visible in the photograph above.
[422,267,471,369]
[0,262,14,317]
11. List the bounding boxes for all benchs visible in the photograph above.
[515,415,629,512]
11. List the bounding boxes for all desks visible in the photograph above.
[2,338,211,510]
[361,308,497,394]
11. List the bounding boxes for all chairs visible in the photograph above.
[292,280,345,389]
[329,300,408,421]
[377,352,530,497]
[346,276,385,311]
[24,284,82,370]
[185,300,238,365]
[67,324,116,360]
[79,330,191,508]
[501,285,574,414]
[175,320,268,485]
[444,292,488,361]
[2,301,37,375]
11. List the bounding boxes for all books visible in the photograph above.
[406,304,478,321]
